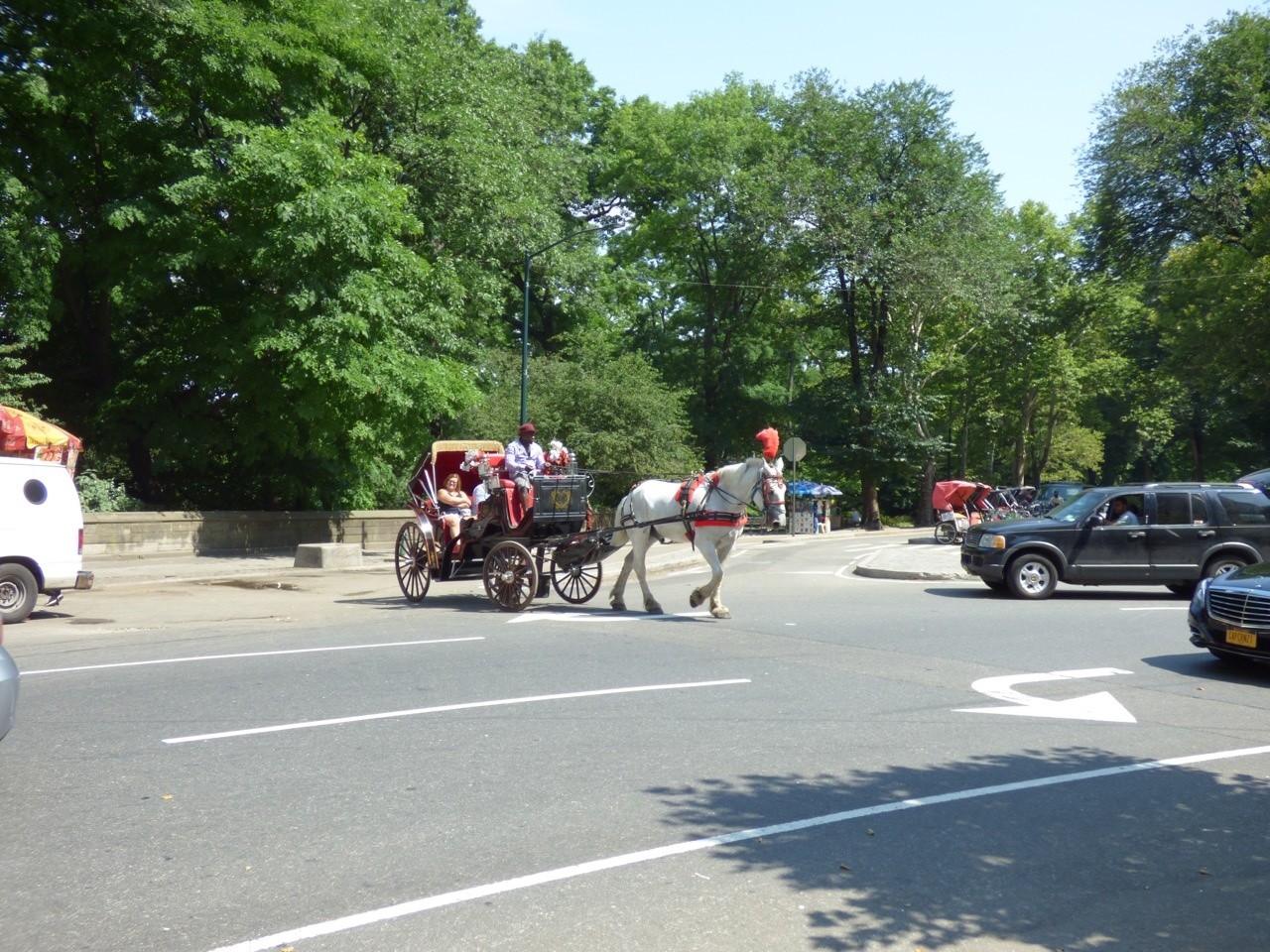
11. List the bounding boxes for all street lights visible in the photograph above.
[517,221,624,421]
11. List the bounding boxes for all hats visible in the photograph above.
[519,423,537,434]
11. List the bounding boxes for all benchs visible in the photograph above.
[424,496,473,529]
[488,454,533,488]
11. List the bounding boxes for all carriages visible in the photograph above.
[392,440,790,620]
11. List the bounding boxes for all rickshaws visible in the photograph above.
[932,480,1060,545]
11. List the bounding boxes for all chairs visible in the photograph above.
[940,503,969,522]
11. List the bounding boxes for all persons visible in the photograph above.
[1049,491,1063,504]
[437,474,471,549]
[505,424,550,516]
[474,479,490,509]
[815,500,823,516]
[848,506,861,523]
[1098,496,1140,525]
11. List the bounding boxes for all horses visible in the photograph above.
[609,454,786,619]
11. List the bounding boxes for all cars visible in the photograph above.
[1188,561,1270,674]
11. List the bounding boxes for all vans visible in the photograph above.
[0,457,95,627]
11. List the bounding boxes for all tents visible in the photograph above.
[0,402,82,478]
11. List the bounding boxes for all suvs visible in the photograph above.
[959,481,1270,600]
[1030,483,1099,510]
[1233,468,1270,499]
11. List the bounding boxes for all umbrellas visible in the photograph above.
[813,482,843,499]
[785,479,818,499]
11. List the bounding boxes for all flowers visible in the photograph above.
[547,440,567,462]
[460,450,490,472]
